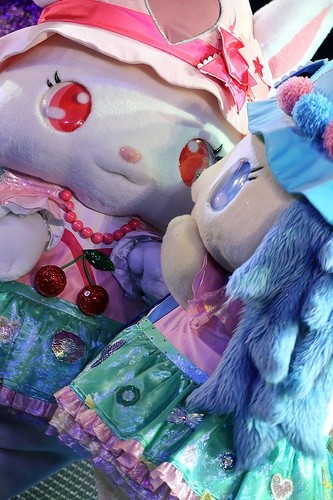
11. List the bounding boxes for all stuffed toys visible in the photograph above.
[0,0,332,500]
